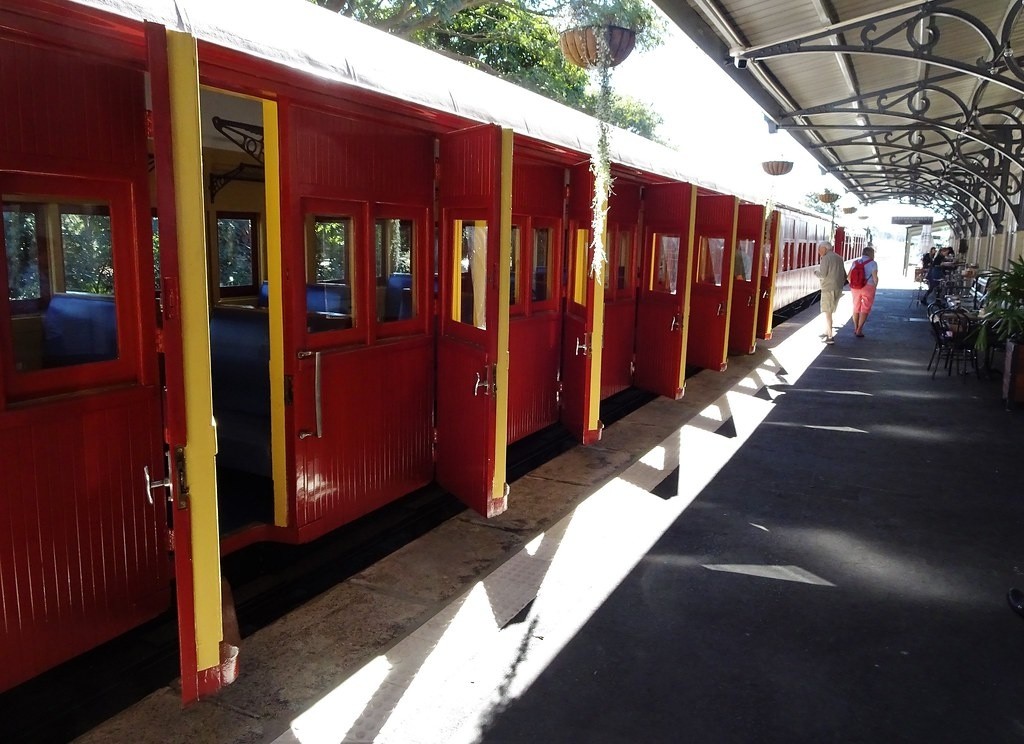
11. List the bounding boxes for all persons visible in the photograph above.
[735,247,752,281]
[813,242,844,344]
[846,247,878,336]
[921,246,957,306]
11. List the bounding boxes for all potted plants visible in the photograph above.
[963,254,1024,412]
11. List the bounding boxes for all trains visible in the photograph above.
[0,0,872,698]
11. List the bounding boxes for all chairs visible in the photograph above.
[927,298,1007,381]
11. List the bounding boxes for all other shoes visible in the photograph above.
[854,331,864,337]
[819,333,828,337]
[821,337,835,344]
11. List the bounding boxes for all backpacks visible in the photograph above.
[849,259,873,289]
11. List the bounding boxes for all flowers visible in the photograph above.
[513,0,674,286]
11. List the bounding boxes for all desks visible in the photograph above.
[943,313,979,321]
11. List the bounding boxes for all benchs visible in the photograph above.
[42,292,116,367]
[381,273,439,321]
[942,274,991,310]
[210,305,352,478]
[257,279,351,313]
